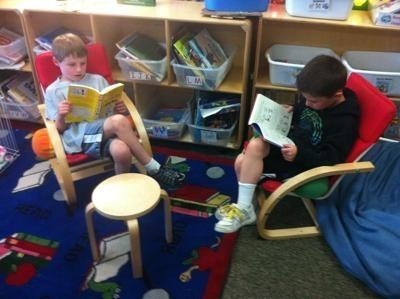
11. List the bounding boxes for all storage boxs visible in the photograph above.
[343,50,400,97]
[114,42,167,83]
[186,109,238,147]
[0,100,39,122]
[263,42,341,86]
[0,36,24,67]
[203,0,270,14]
[285,0,354,21]
[171,43,237,90]
[140,91,190,139]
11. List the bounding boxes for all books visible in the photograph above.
[187,89,241,142]
[146,106,185,137]
[248,93,296,149]
[0,22,92,118]
[65,83,124,123]
[118,31,165,81]
[172,28,227,88]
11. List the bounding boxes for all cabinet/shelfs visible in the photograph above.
[249,6,400,139]
[0,1,252,150]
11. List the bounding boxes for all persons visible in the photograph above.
[214,54,363,234]
[45,34,186,184]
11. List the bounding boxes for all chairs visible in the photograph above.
[34,43,154,204]
[244,73,396,240]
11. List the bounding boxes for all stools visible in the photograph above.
[84,173,175,278]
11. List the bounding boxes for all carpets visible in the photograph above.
[0,120,238,298]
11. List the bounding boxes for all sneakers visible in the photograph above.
[214,203,257,234]
[146,164,187,188]
